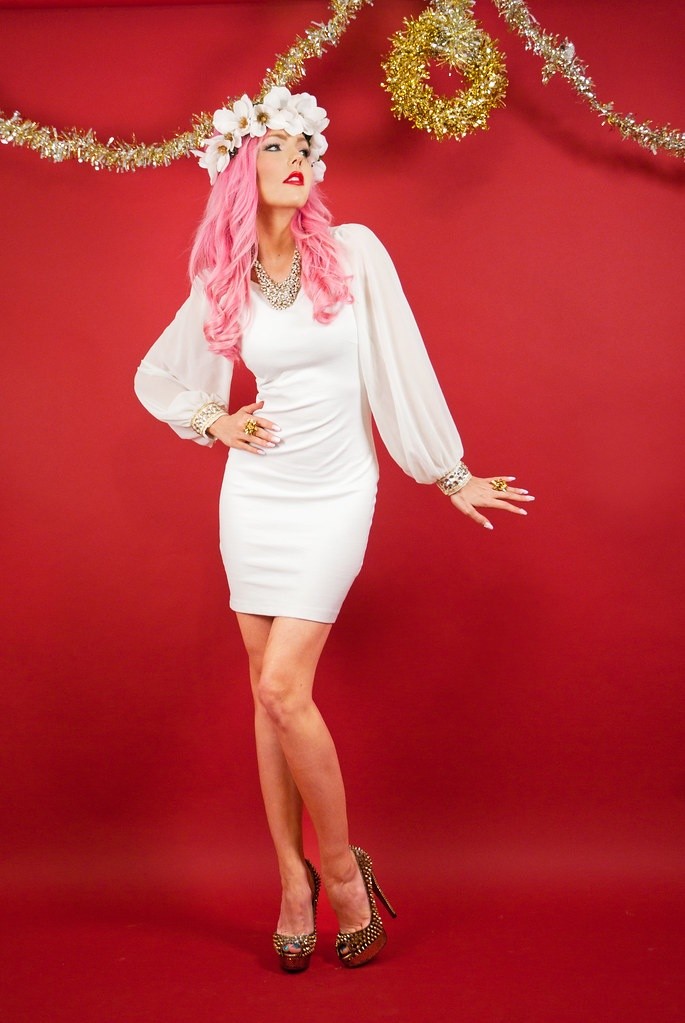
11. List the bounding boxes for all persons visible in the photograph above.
[134,87,534,969]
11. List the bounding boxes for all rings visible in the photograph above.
[490,479,508,492]
[244,420,258,434]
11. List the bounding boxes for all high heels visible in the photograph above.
[335,844,399,967]
[273,858,320,971]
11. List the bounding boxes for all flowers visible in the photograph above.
[190,86,330,185]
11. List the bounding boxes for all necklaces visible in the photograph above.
[254,248,302,311]
